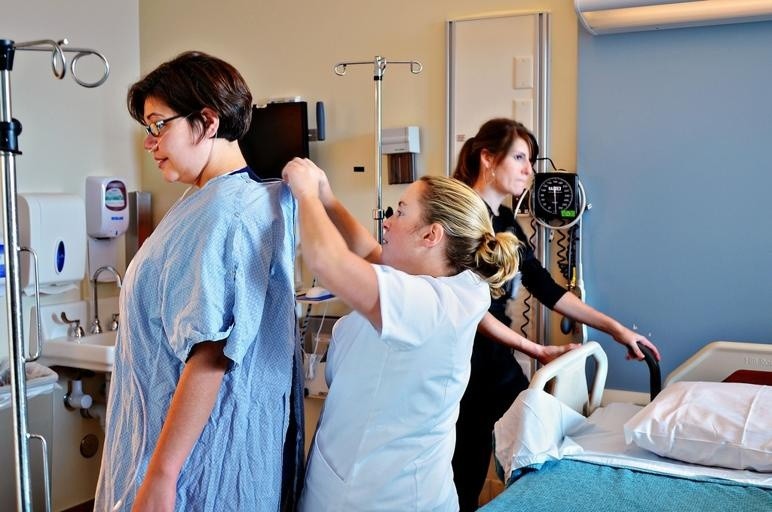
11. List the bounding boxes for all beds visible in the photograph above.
[466,339,772,511]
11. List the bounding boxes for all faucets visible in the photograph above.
[91,260,123,334]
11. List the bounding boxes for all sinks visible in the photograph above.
[28,300,118,373]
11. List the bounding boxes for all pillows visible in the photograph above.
[619,380,772,474]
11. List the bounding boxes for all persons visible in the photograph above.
[282,156,526,511]
[453,118,660,511]
[90,50,300,512]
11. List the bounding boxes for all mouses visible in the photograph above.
[305,286,330,299]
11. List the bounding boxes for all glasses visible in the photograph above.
[145,115,184,137]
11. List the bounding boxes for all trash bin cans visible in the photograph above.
[0,361,60,510]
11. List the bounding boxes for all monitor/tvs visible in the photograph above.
[238,102,310,181]
